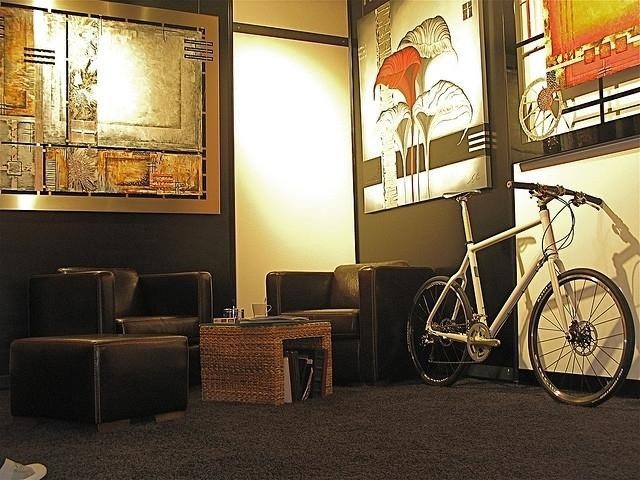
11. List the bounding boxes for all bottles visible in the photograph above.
[223,305,245,318]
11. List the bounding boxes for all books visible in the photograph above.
[281,350,330,405]
[240,315,310,326]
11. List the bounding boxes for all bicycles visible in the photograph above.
[407,180,635,406]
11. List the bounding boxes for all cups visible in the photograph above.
[252,304,272,317]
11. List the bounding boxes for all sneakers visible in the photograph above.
[0,458,49,480]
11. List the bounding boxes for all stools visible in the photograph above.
[10,332,189,435]
